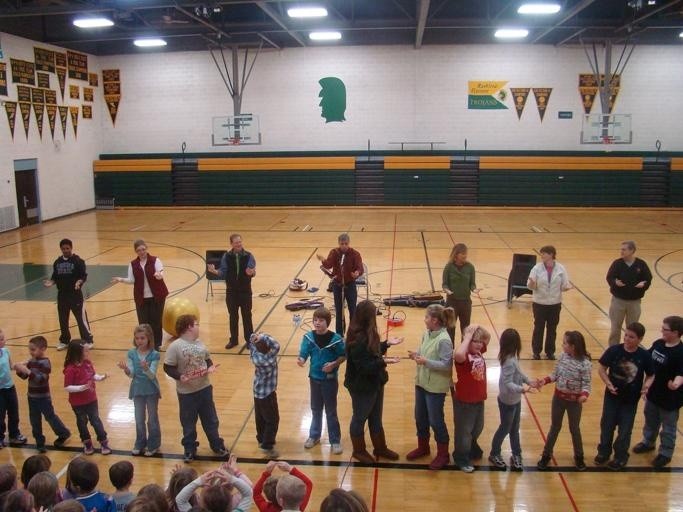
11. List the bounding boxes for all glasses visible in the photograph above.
[662,326,675,332]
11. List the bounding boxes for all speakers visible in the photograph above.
[205,250,229,280]
[512,254,537,286]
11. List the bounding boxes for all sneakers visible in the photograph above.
[534,350,556,361]
[132,446,159,457]
[453,442,672,474]
[258,442,279,458]
[82,439,111,455]
[224,340,238,349]
[183,448,197,463]
[0,432,72,453]
[304,436,343,455]
[57,342,70,350]
[212,445,229,457]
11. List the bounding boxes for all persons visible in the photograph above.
[606,241,652,346]
[109,239,168,352]
[527,245,574,360]
[207,234,256,349]
[232,263,236,265]
[441,244,483,350]
[43,239,94,351]
[319,234,364,337]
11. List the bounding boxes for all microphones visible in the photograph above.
[341,254,346,266]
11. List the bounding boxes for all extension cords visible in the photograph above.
[377,311,382,315]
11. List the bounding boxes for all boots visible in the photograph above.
[406,435,450,471]
[350,430,399,468]
